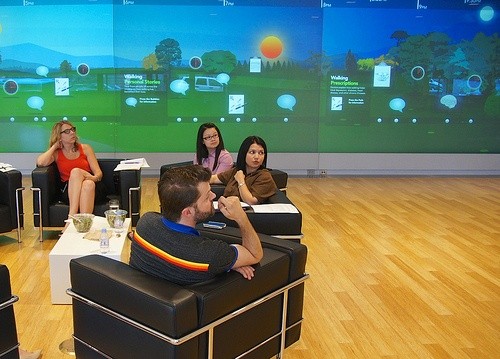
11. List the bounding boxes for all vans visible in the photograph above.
[179,76,222,92]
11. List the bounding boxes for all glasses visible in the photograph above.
[60,127,76,134]
[203,134,218,141]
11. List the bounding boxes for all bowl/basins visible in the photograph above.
[104,209,128,228]
[72,213,95,233]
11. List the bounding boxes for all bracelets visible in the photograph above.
[238,182,245,188]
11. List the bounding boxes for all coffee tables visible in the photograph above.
[49,216,132,305]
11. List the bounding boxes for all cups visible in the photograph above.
[109,199,119,209]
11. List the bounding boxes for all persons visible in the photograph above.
[206,136,278,204]
[129,165,264,287]
[36,120,103,236]
[193,122,234,176]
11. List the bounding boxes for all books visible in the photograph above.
[212,199,249,208]
[250,203,299,214]
[113,157,150,172]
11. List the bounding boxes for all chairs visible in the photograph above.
[30,158,141,243]
[0,169,25,244]
[66,223,308,359]
[0,263,20,359]
[160,161,304,243]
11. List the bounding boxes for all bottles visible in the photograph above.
[114,211,123,233]
[99,228,108,254]
